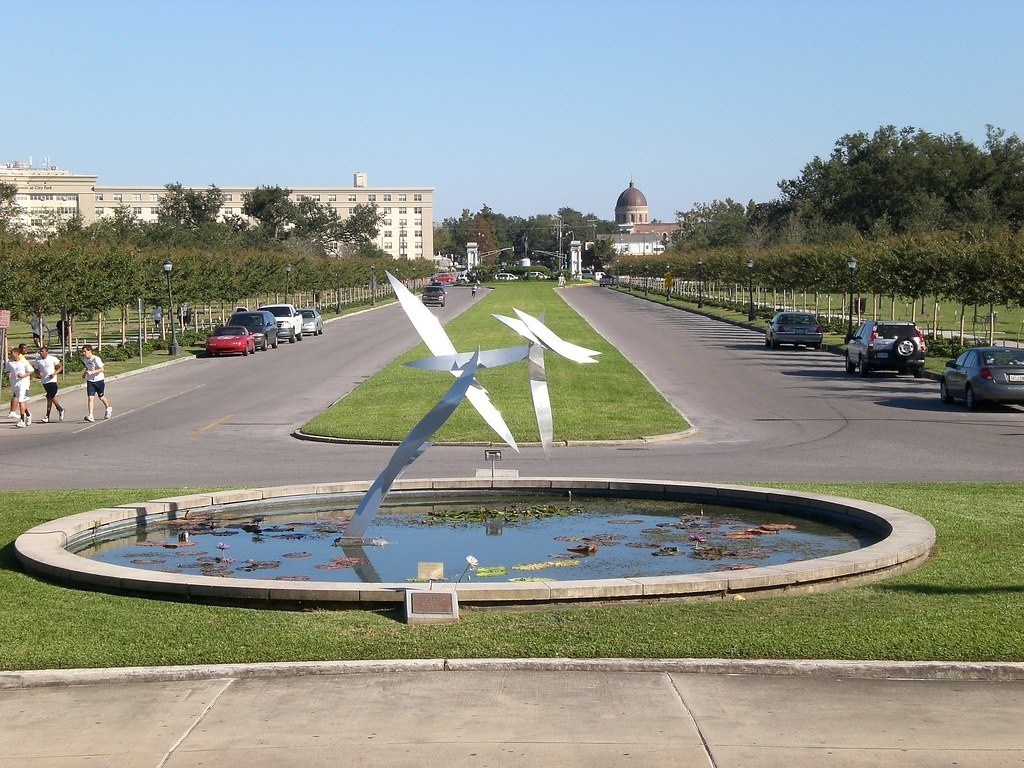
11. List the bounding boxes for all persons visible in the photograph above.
[4,340,35,429]
[56,315,70,354]
[28,310,45,349]
[82,343,112,423]
[471,284,477,298]
[151,304,164,329]
[34,347,66,424]
[175,299,192,331]
[557,273,566,287]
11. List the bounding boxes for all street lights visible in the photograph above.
[284,263,292,303]
[845,256,856,344]
[698,258,703,308]
[616,259,671,301]
[163,259,179,355]
[370,263,416,305]
[747,259,756,321]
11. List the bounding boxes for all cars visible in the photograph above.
[940,346,1024,408]
[434,274,454,282]
[765,311,823,351]
[295,309,323,336]
[205,326,255,356]
[431,282,444,289]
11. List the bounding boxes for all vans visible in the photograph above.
[496,272,548,280]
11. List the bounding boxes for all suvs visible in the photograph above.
[258,305,303,343]
[228,311,277,351]
[458,273,470,283]
[845,319,926,378]
[421,286,446,307]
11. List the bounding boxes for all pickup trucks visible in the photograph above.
[600,275,613,287]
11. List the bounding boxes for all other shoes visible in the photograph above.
[103,407,113,419]
[26,412,32,426]
[58,409,65,421]
[84,414,95,422]
[41,415,50,422]
[15,419,25,428]
[6,411,17,418]
